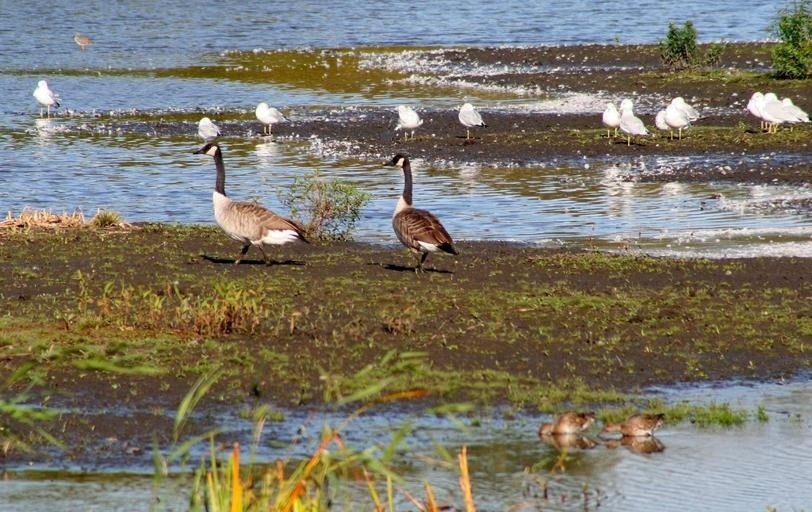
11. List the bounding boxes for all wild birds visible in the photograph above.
[33,80,60,118]
[254,101,291,135]
[600,93,708,148]
[394,104,424,141]
[197,116,223,141]
[747,90,812,134]
[457,102,490,142]
[71,31,96,50]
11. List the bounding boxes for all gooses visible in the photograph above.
[539,435,667,454]
[381,153,460,276]
[193,142,311,266]
[538,410,668,435]
